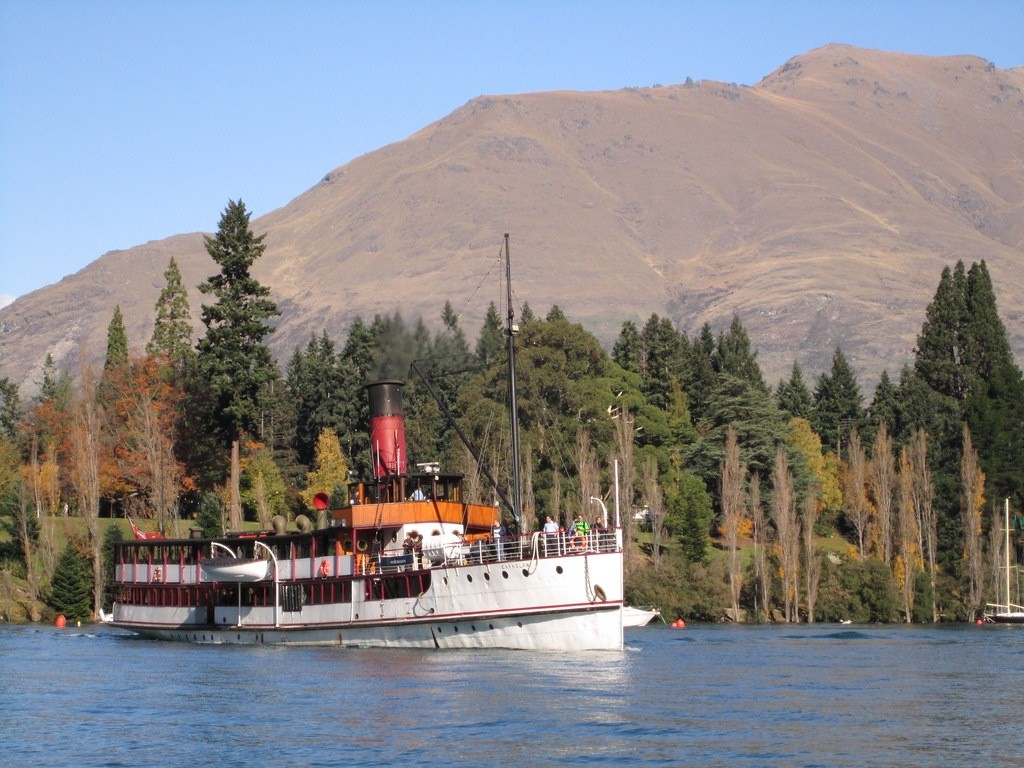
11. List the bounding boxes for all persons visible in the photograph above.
[543,515,609,554]
[349,489,361,504]
[490,519,507,563]
[410,485,433,500]
[402,529,472,571]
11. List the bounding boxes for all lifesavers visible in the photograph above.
[154,567,162,581]
[569,534,584,552]
[320,560,330,576]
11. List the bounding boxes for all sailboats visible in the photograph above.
[979,499,1024,623]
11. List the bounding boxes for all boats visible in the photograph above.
[422,542,463,561]
[198,553,269,583]
[371,552,419,571]
[99,235,625,652]
[623,605,661,627]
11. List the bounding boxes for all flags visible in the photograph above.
[129,518,166,540]
